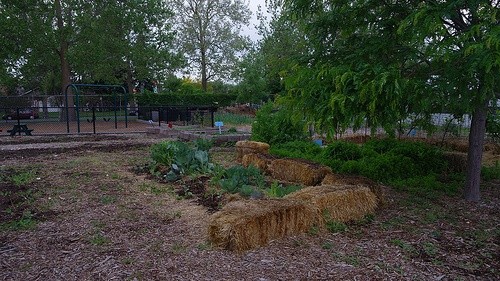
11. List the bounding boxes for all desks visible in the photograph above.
[6,124,34,136]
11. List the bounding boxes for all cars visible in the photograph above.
[2,109,39,119]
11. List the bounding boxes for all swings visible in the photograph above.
[102,86,111,122]
[86,86,96,123]
